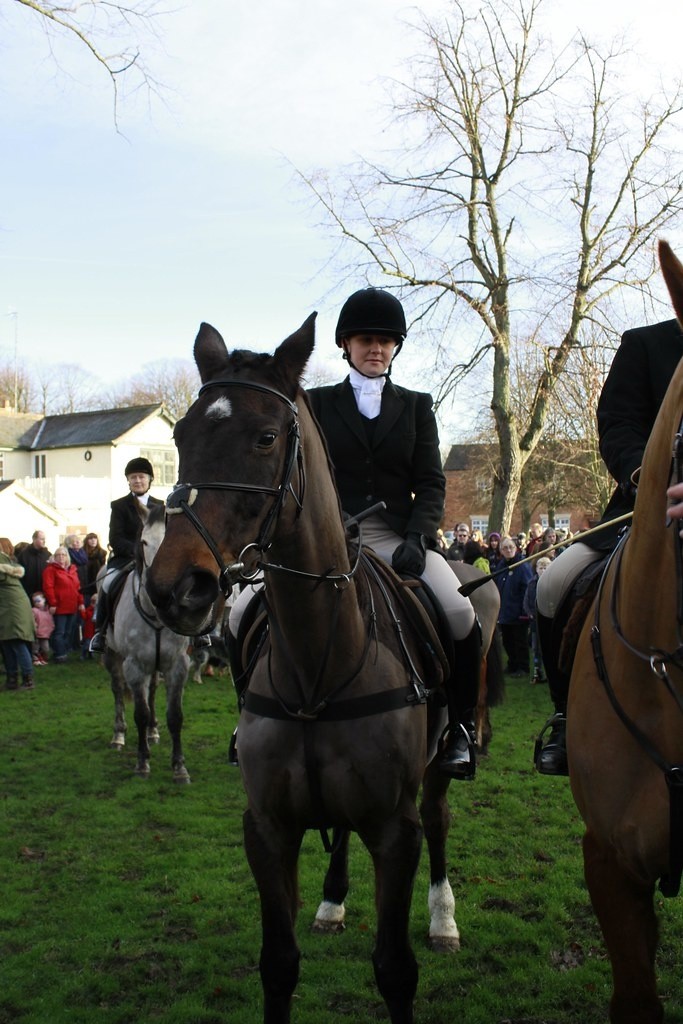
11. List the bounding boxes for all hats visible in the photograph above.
[457,522,469,532]
[489,533,500,540]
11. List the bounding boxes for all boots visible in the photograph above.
[91,585,109,650]
[443,612,483,780]
[225,619,248,714]
[536,599,566,772]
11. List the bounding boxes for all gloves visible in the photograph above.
[392,534,426,579]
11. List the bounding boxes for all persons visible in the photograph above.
[435,524,588,684]
[536,320,683,771]
[92,457,210,651]
[192,623,220,684]
[224,287,484,772]
[0,529,107,693]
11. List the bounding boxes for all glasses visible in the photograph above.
[457,534,467,537]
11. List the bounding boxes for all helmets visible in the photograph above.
[336,288,407,347]
[125,458,154,477]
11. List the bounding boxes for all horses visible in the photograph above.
[144,310,501,1024]
[102,494,202,785]
[563,240,683,1024]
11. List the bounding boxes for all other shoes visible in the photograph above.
[33,660,44,666]
[529,674,543,685]
[6,672,18,688]
[23,674,34,689]
[503,667,515,675]
[41,660,48,665]
[511,670,529,678]
